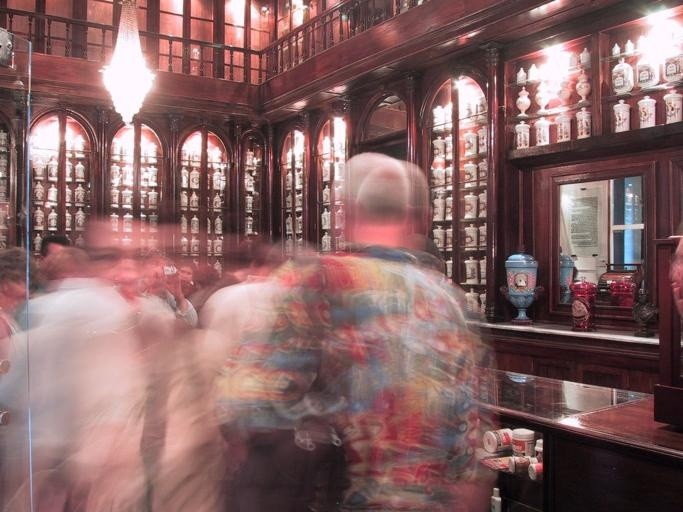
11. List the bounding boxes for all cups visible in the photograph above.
[0,127,11,247]
[503,253,538,321]
[557,254,572,305]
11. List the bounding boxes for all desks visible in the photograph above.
[474,366,683,512]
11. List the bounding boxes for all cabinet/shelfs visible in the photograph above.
[427,108,490,289]
[177,155,232,256]
[277,155,306,255]
[510,58,597,161]
[102,149,169,250]
[605,43,683,138]
[315,143,346,255]
[21,148,92,249]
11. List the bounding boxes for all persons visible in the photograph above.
[0,152,497,512]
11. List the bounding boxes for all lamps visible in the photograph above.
[96,1,157,129]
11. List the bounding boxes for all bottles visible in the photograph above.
[566,274,598,330]
[514,26,682,153]
[482,427,543,481]
[426,98,490,319]
[609,280,634,307]
[27,130,346,279]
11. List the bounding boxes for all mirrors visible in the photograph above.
[548,160,657,321]
